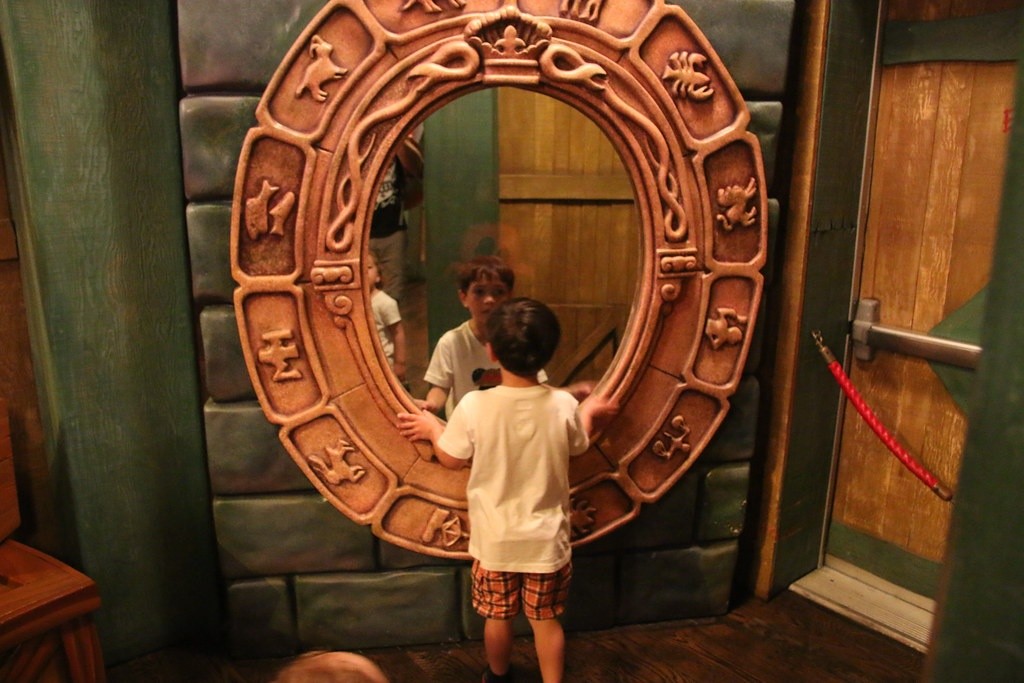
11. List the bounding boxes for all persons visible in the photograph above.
[367,133,424,302]
[397,297,620,683]
[366,251,413,393]
[414,254,595,423]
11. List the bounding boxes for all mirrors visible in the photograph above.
[229,1,768,559]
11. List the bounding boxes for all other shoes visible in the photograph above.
[481,663,514,683]
